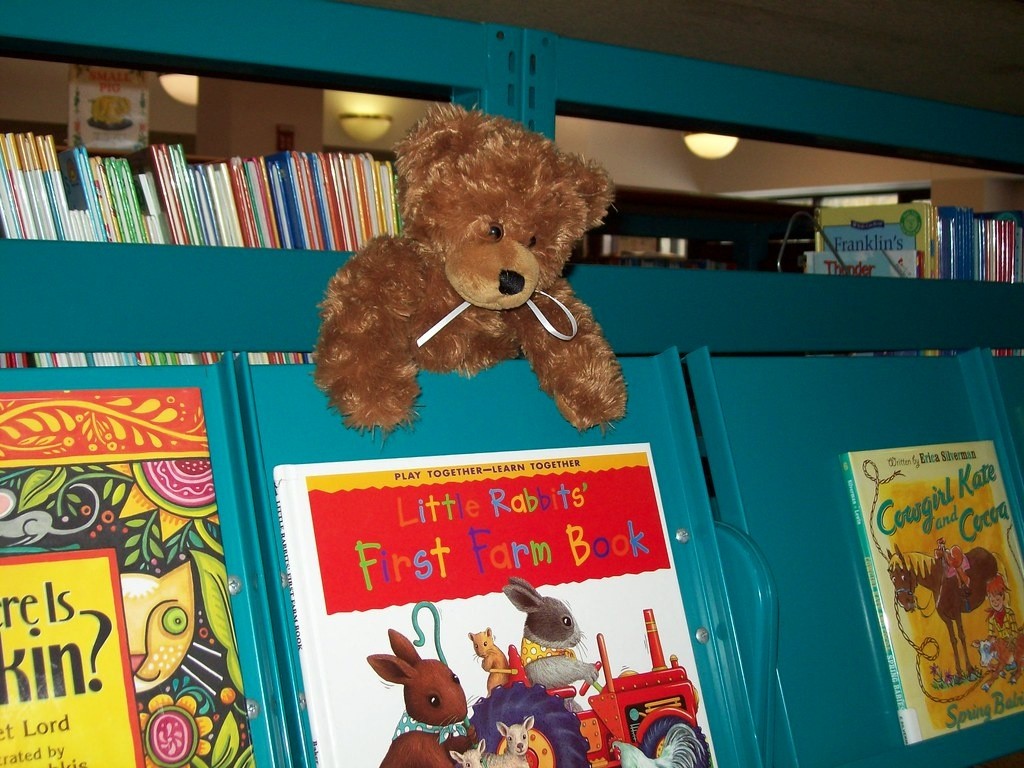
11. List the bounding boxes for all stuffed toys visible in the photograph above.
[312,100,629,438]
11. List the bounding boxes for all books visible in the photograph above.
[274,443,716,768]
[842,440,1023,748]
[0,132,404,364]
[584,253,742,270]
[804,202,1024,356]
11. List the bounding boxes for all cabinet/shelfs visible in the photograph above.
[0,1,1024,768]
